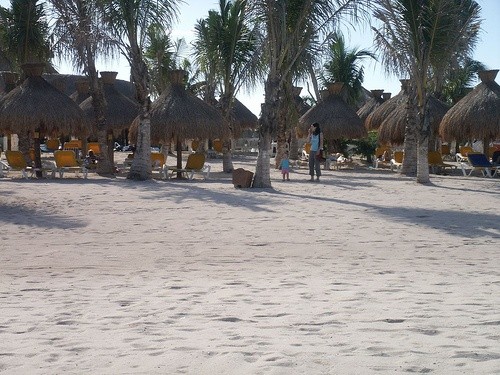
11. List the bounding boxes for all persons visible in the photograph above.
[306,122,323,182]
[84,150,100,169]
[277,152,293,182]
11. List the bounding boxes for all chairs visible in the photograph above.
[0,139,103,180]
[288,148,403,172]
[456,145,500,178]
[427,153,456,174]
[125,139,223,182]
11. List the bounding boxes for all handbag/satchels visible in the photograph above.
[318,150,324,162]
